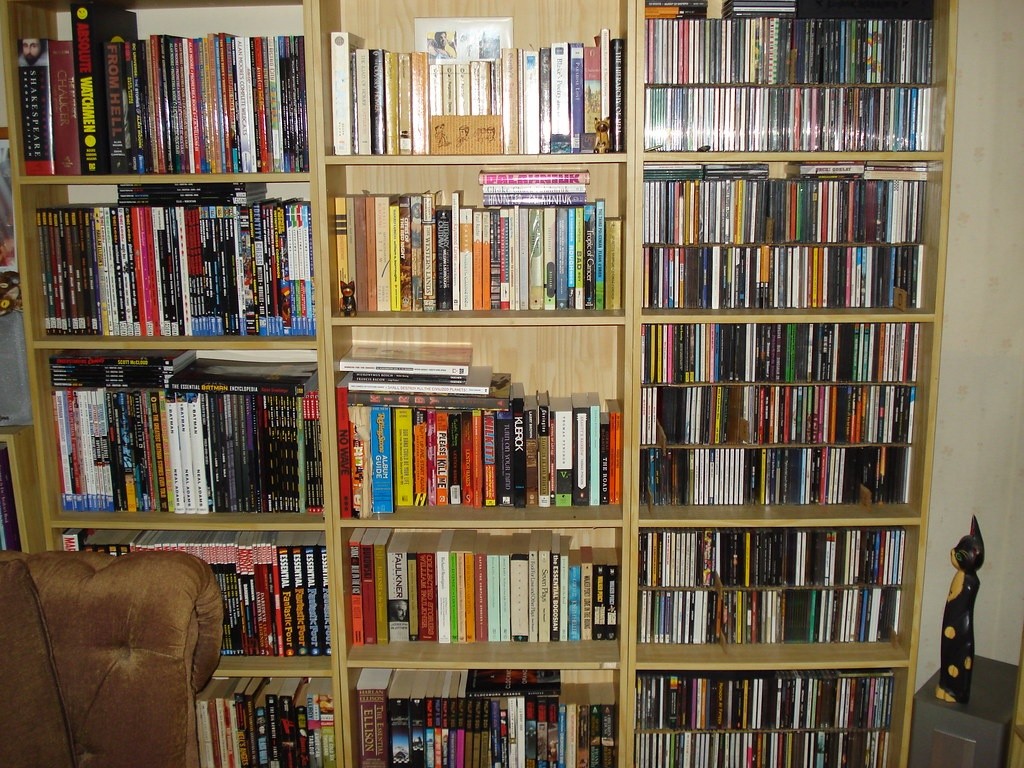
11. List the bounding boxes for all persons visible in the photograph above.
[18,38,48,66]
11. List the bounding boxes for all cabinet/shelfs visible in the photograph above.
[0,0,346,768]
[625,0,961,767]
[311,0,635,768]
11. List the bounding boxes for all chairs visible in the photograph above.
[0,549,225,768]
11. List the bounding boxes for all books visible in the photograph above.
[329,27,624,767]
[16,2,335,768]
[632,0,934,768]
